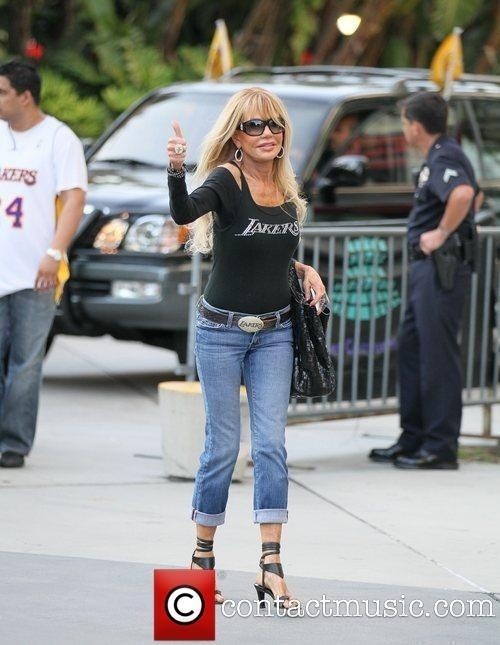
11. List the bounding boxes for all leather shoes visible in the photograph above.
[394,454,458,471]
[1,452,24,468]
[370,445,419,460]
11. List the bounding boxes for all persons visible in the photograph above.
[1,61,88,467]
[370,90,484,469]
[312,116,389,183]
[167,87,330,610]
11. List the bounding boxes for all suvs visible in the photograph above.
[44,62,500,386]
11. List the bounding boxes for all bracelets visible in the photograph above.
[439,224,451,238]
[165,166,186,178]
[45,248,63,262]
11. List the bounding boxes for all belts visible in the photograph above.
[196,298,294,332]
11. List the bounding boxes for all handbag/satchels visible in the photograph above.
[288,291,336,399]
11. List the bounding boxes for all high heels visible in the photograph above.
[191,537,224,605]
[255,543,298,610]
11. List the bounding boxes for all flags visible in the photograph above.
[204,18,234,86]
[429,26,464,90]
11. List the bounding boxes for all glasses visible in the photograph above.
[236,117,285,137]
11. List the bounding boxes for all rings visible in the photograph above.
[174,143,183,152]
